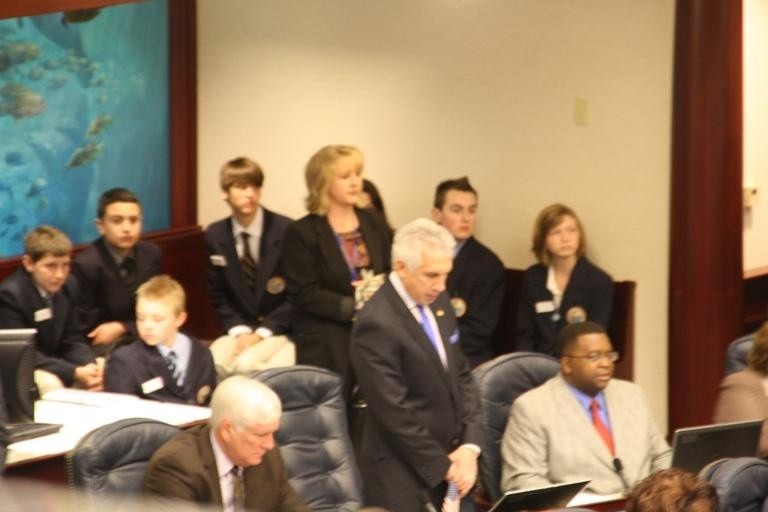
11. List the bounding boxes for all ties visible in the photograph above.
[163,352,184,389]
[120,256,136,296]
[591,399,615,458]
[42,292,55,317]
[240,233,258,295]
[417,305,437,356]
[230,466,245,511]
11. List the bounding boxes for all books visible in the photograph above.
[208,333,297,377]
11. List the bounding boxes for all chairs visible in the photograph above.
[63,417,184,500]
[725,332,756,376]
[472,352,561,501]
[244,364,367,512]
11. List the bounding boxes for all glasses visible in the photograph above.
[558,349,619,364]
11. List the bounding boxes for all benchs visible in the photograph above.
[0,225,225,347]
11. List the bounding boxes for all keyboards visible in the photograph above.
[4,422,63,443]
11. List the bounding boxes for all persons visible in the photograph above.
[285,146,394,385]
[66,189,164,357]
[138,376,312,512]
[104,274,217,407]
[359,181,385,228]
[623,470,719,511]
[714,318,768,459]
[513,198,614,360]
[201,156,297,349]
[443,495,464,512]
[425,175,508,373]
[1,228,104,395]
[499,322,674,498]
[347,218,487,512]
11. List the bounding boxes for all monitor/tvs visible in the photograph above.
[671,418,765,477]
[488,479,592,512]
[0,326,39,425]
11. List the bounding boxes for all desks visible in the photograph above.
[4,388,211,486]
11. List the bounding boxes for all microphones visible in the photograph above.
[613,458,631,490]
